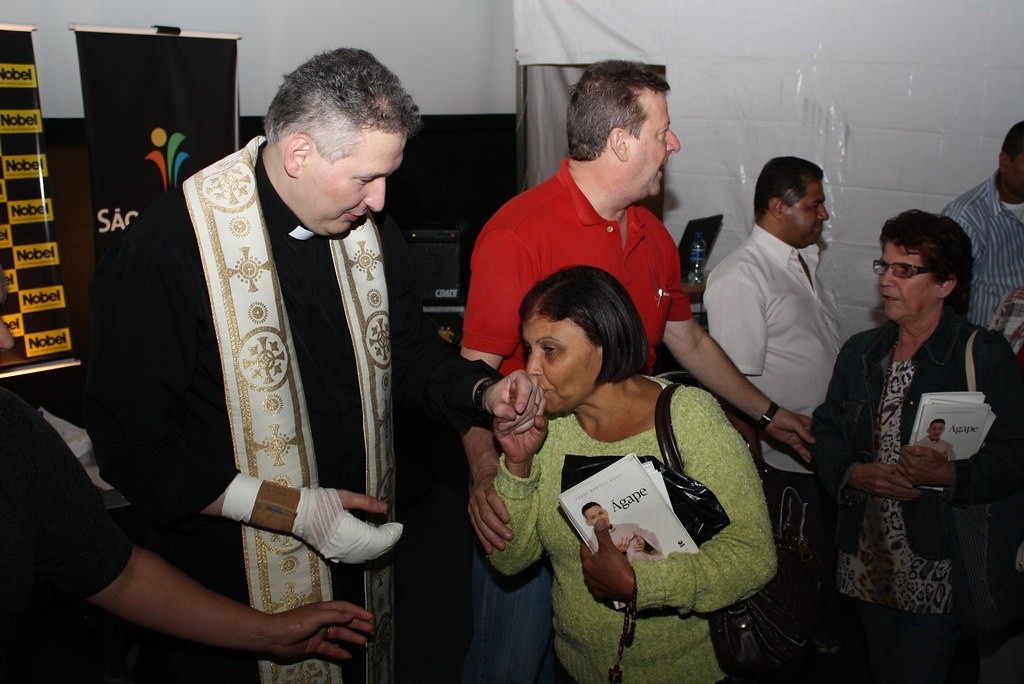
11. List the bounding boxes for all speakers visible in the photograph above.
[402,228,472,306]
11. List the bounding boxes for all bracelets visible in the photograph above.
[474,378,498,409]
[607,574,636,684]
[756,402,779,429]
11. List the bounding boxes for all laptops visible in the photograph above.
[677,214,724,282]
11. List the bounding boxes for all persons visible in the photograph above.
[459,59,816,684]
[703,154,873,684]
[918,416,955,461]
[582,502,662,564]
[941,121,1023,367]
[1,260,374,684]
[86,45,545,684]
[808,209,1024,684]
[485,265,779,684]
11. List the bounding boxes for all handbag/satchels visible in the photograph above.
[953,326,1023,636]
[654,379,821,684]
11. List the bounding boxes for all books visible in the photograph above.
[908,390,997,491]
[558,451,698,610]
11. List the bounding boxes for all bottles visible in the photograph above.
[689,231,707,283]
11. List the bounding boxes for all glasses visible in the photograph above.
[873,259,933,279]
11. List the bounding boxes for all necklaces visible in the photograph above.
[874,336,915,449]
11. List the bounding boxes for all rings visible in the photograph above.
[534,403,539,408]
[326,627,330,634]
